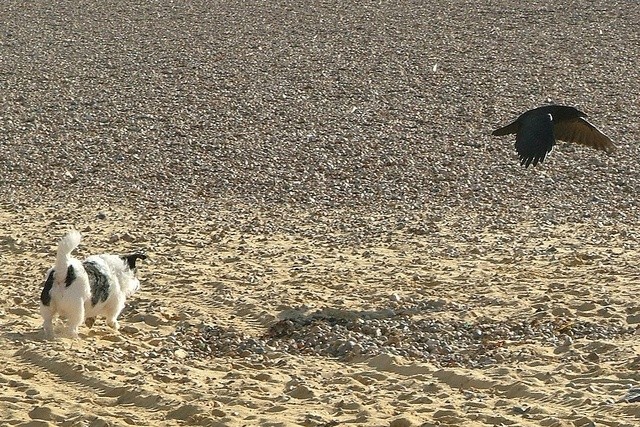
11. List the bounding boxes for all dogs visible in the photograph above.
[39,229,149,342]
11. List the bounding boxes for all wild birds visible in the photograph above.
[491,104,619,168]
